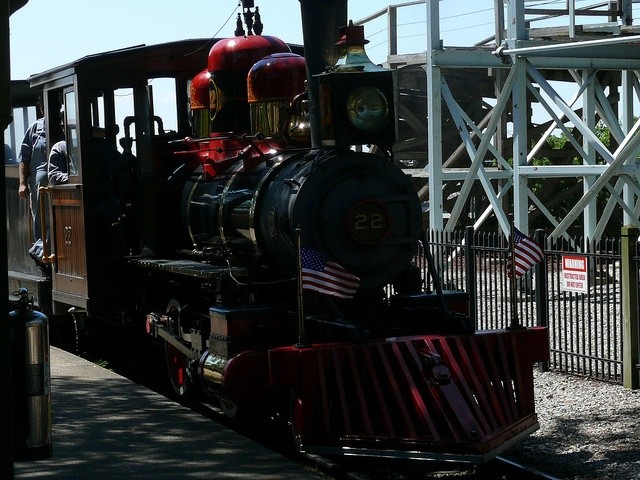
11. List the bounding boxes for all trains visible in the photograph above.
[0,1,550,480]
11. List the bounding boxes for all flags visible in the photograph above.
[298,249,362,301]
[505,227,547,286]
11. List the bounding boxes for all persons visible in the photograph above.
[15,100,51,272]
[47,119,79,197]
